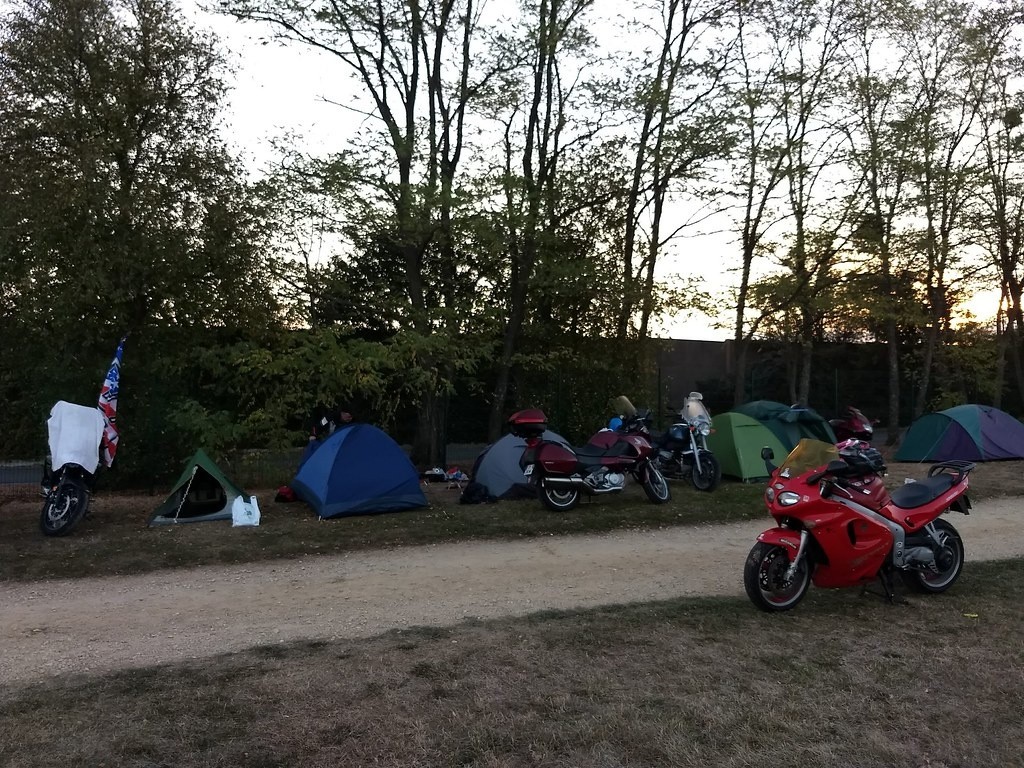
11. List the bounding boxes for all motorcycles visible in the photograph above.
[506,408,670,510]
[744,437,978,610]
[618,392,722,492]
[35,402,119,536]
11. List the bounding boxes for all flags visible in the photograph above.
[97,334,128,468]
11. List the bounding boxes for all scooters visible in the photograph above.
[829,406,876,444]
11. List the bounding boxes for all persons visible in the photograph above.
[309,406,357,443]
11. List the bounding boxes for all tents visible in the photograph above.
[891,404,1024,463]
[458,426,576,504]
[146,446,257,527]
[289,421,431,519]
[703,399,840,483]
